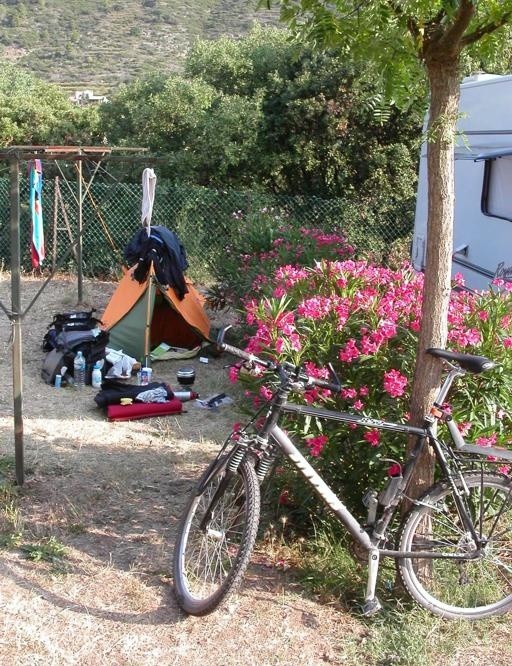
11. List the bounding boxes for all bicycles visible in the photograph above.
[172,325,512,622]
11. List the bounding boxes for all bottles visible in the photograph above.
[173,391,199,401]
[92,364,102,388]
[73,351,86,388]
[55,374,62,388]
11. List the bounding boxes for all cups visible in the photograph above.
[141,367,152,386]
